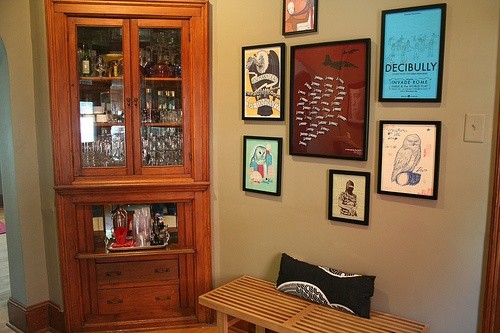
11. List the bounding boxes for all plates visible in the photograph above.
[107,232,170,252]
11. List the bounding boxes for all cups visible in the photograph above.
[82,128,125,167]
[142,127,184,167]
[106,110,118,123]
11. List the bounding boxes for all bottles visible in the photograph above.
[141,85,183,124]
[112,205,167,246]
[140,29,182,77]
[77,38,120,77]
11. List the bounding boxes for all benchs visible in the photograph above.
[198,275,431,333]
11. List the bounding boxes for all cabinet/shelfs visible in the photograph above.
[44,0,215,333]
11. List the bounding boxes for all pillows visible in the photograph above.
[274,253,377,319]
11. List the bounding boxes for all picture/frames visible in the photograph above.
[288,38,372,162]
[242,135,283,196]
[241,42,285,122]
[377,120,442,200]
[377,2,448,103]
[328,169,372,227]
[282,0,318,36]
